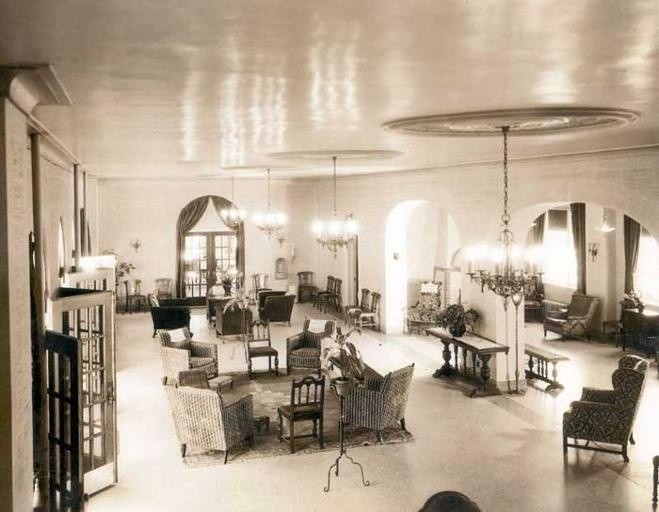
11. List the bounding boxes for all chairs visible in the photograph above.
[155,278,174,298]
[286,316,337,378]
[166,385,254,465]
[406,280,442,336]
[525,298,541,323]
[337,362,415,452]
[276,375,326,454]
[563,354,649,461]
[146,294,191,338]
[124,278,147,315]
[419,491,481,512]
[178,370,210,389]
[246,318,279,379]
[257,285,297,326]
[158,327,218,385]
[344,288,370,327]
[353,292,380,332]
[312,275,335,310]
[318,278,343,313]
[296,271,316,302]
[215,298,254,343]
[602,297,644,347]
[542,289,600,342]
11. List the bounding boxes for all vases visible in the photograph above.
[449,321,466,337]
[221,272,236,295]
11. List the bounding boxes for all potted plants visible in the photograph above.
[325,344,360,397]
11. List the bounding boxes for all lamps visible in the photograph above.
[313,156,358,259]
[220,176,248,233]
[595,205,615,234]
[130,236,140,253]
[277,230,286,247]
[465,126,545,299]
[256,170,288,236]
[587,240,598,262]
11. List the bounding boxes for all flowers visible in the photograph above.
[436,304,478,330]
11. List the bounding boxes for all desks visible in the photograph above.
[425,326,509,399]
[206,295,231,324]
[623,308,659,351]
[524,343,568,392]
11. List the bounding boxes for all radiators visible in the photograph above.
[541,299,568,320]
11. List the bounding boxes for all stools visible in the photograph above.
[252,415,269,433]
